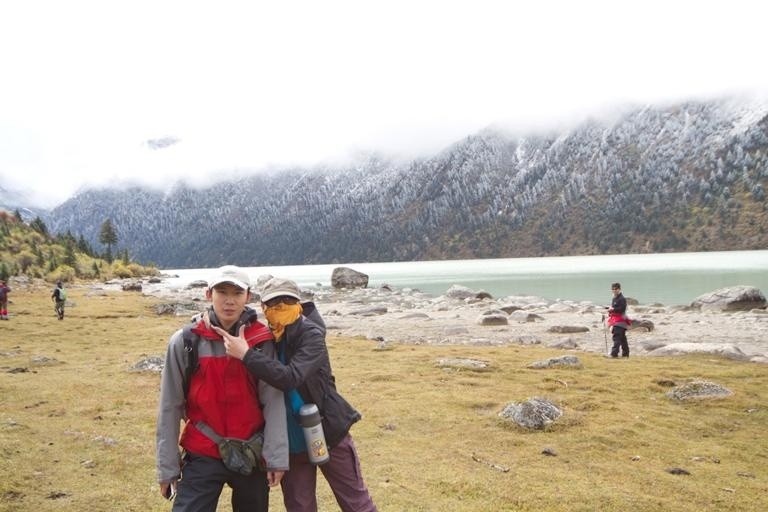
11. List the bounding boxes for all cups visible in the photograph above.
[298,403,330,466]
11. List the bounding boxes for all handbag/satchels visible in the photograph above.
[218,434,265,476]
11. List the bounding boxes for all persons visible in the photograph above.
[52,279,66,321]
[604,280,630,359]
[154,266,291,511]
[0,279,12,321]
[208,276,381,511]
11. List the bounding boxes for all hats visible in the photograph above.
[262,277,300,304]
[209,265,251,291]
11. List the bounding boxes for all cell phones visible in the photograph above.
[167,485,176,502]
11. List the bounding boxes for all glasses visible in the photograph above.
[265,295,298,308]
